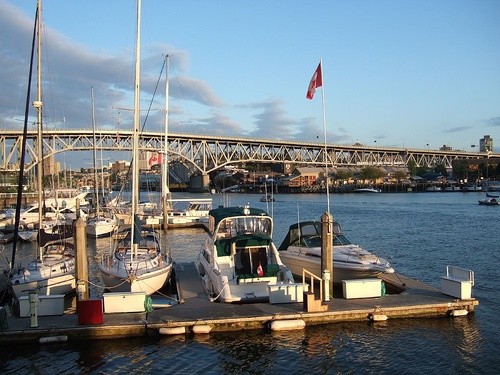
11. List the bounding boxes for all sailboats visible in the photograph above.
[97,0,174,296]
[0,0,76,303]
[259,178,276,203]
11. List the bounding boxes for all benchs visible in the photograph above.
[341,278,383,299]
[103,292,146,313]
[440,266,475,300]
[18,295,65,316]
[268,283,310,304]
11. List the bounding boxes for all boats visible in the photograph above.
[194,170,295,304]
[0,185,212,243]
[477,192,500,205]
[426,180,500,192]
[353,185,382,193]
[277,201,395,288]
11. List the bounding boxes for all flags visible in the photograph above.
[257,264,263,276]
[306,64,322,100]
[149,154,161,165]
